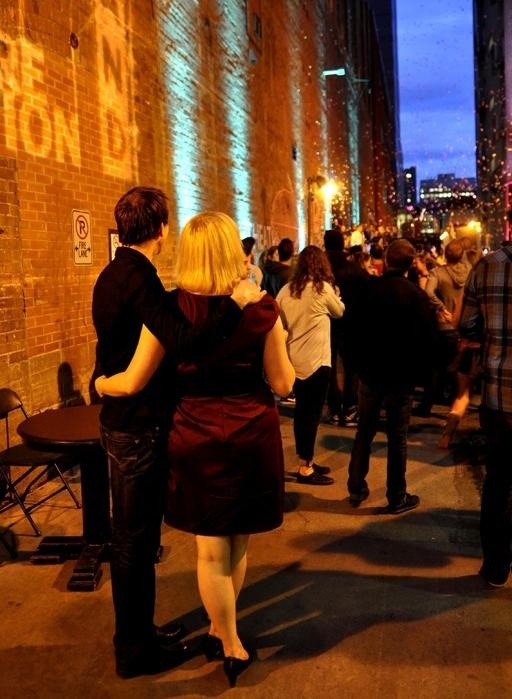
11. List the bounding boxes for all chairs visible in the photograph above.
[0,386,80,537]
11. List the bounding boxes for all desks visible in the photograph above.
[15,401,164,596]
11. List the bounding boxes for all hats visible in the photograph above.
[323,229,345,254]
[240,237,256,257]
[384,237,417,273]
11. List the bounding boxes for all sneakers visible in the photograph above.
[327,411,340,425]
[387,495,420,514]
[347,488,372,508]
[344,407,360,427]
[481,563,511,586]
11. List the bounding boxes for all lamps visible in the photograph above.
[308,172,331,200]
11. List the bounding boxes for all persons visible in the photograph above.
[243,208,491,450]
[455,208,512,589]
[92,187,267,678]
[95,209,295,688]
[276,245,345,485]
[343,238,440,514]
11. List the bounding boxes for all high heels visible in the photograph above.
[221,652,252,687]
[200,635,224,662]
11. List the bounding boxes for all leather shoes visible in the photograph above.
[296,470,334,485]
[312,463,331,475]
[151,622,186,644]
[115,639,192,680]
